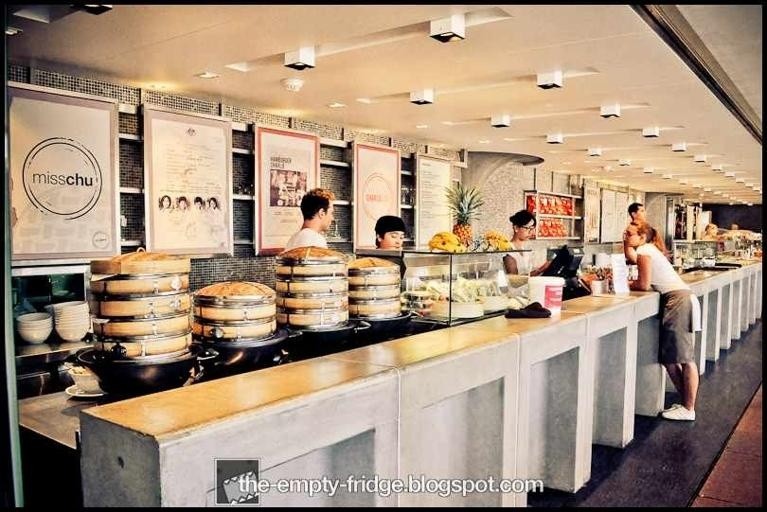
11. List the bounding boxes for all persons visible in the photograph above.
[193,196,204,211]
[373,216,407,249]
[701,223,719,241]
[159,195,172,213]
[500,209,551,296]
[176,196,189,210]
[284,188,334,250]
[622,220,701,422]
[622,202,645,265]
[207,197,219,210]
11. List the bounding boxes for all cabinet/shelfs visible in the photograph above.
[16,241,763,508]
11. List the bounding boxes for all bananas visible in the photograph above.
[482,233,515,252]
[430,233,469,252]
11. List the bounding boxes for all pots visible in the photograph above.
[277,320,372,364]
[76,341,204,398]
[347,309,414,347]
[193,325,292,376]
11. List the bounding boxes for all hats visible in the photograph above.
[376,216,406,232]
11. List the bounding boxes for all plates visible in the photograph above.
[65,385,107,400]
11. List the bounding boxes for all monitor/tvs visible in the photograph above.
[540,244,586,276]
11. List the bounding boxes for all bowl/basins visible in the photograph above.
[15,301,91,345]
[67,366,101,393]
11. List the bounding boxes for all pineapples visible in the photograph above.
[446,180,484,246]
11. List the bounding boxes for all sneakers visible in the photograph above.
[661,403,696,421]
